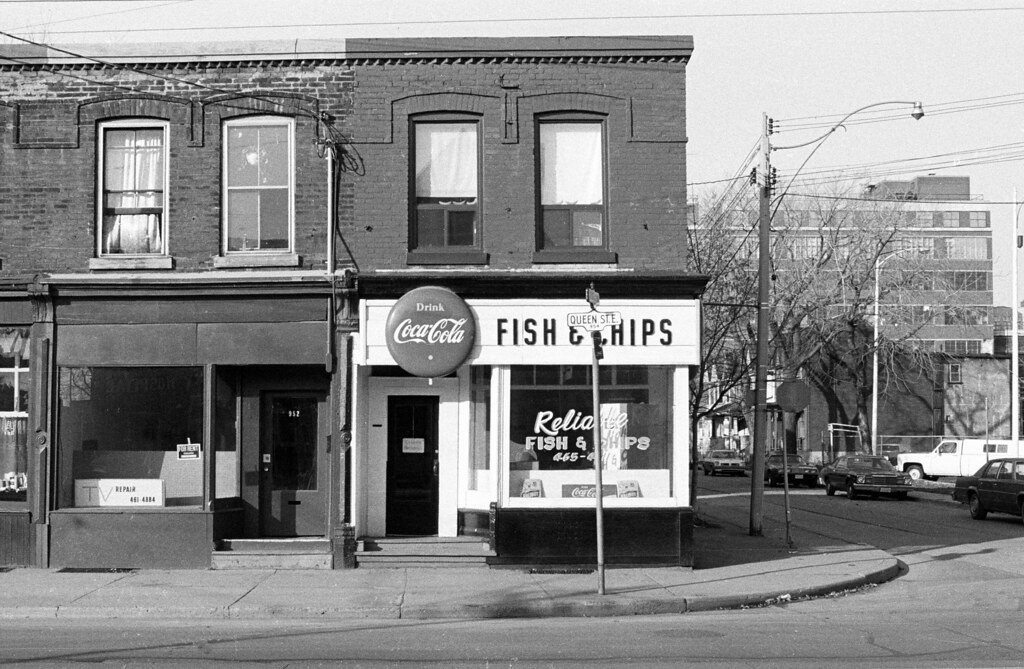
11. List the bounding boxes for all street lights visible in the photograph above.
[749,101,926,539]
[872,246,931,458]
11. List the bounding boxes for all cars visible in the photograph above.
[764,452,820,488]
[952,457,1024,525]
[689,447,751,470]
[703,449,746,477]
[819,454,913,500]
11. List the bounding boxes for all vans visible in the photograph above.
[873,443,911,467]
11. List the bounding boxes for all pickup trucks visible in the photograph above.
[896,439,1024,482]
